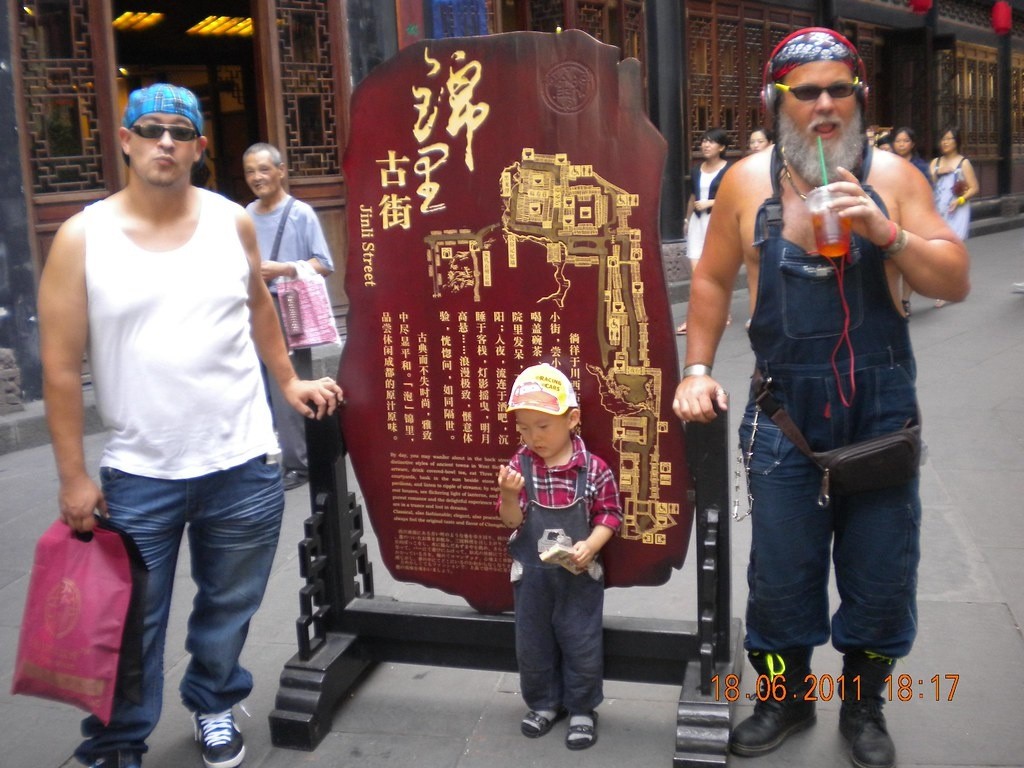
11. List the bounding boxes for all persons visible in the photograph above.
[671,20,970,768]
[497,363,623,749]
[241,141,334,492]
[742,127,775,331]
[35,82,345,768]
[676,128,736,335]
[875,126,930,320]
[926,125,980,309]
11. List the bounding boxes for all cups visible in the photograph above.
[807,182,853,256]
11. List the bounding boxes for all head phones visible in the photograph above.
[760,27,869,115]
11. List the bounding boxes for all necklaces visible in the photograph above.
[777,144,810,202]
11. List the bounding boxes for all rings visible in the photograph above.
[716,388,728,396]
[858,195,868,206]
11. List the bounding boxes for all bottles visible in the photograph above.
[282,291,303,338]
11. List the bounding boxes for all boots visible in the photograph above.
[838,650,901,768]
[729,646,816,753]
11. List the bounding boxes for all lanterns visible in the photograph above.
[992,0,1013,38]
[909,0,932,14]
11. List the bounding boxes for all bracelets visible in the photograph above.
[958,196,966,206]
[683,363,712,377]
[684,218,688,224]
[880,219,908,259]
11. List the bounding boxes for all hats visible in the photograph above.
[507,363,578,415]
[770,31,858,82]
[121,82,202,167]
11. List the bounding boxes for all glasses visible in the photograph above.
[774,76,859,101]
[130,125,199,141]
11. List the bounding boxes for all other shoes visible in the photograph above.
[903,299,910,317]
[677,321,688,335]
[935,299,945,308]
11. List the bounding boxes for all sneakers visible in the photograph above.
[190,704,251,768]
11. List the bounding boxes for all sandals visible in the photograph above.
[521,705,565,738]
[566,710,599,751]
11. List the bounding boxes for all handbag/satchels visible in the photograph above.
[952,178,971,195]
[275,260,336,349]
[749,367,928,506]
[10,514,148,724]
